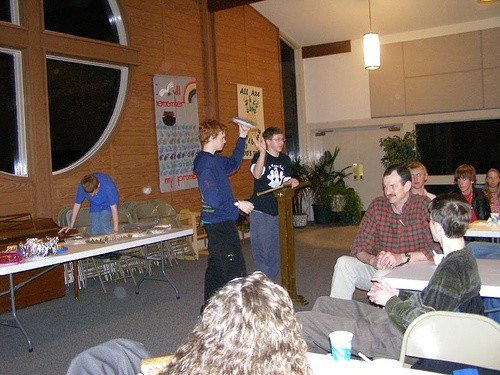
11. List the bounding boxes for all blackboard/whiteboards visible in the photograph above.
[424,183,486,197]
[412,116,500,178]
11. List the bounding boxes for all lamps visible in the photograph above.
[363,0,380,70]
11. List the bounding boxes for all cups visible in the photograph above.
[329,331,353,361]
[490,213,499,227]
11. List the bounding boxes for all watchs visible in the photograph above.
[405,251,411,263]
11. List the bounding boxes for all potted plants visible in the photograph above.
[289,146,362,228]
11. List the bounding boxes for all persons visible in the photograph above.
[193,119,250,315]
[330,163,443,301]
[408,161,500,223]
[58,172,123,260]
[297,192,481,365]
[250,126,302,284]
[156,271,313,375]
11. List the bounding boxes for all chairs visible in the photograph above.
[399,311,500,375]
[59,197,198,294]
[169,209,211,260]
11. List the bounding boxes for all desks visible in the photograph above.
[0,228,193,352]
[464,220,500,243]
[382,260,500,324]
[140,351,446,375]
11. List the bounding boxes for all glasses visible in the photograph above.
[272,138,286,142]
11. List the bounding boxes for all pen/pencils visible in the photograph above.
[399,220,405,226]
